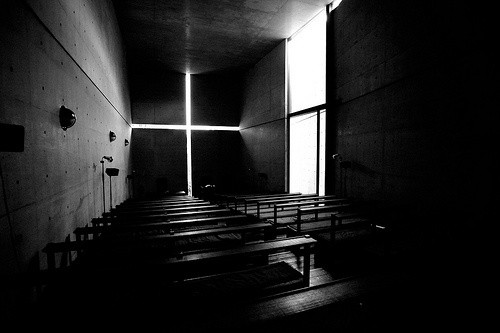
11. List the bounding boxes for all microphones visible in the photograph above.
[103,156,113,162]
[332,153,340,160]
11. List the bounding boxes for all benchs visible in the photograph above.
[27,183,469,331]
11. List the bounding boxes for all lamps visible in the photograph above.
[125,139,129,146]
[110,131,116,142]
[59,105,76,131]
